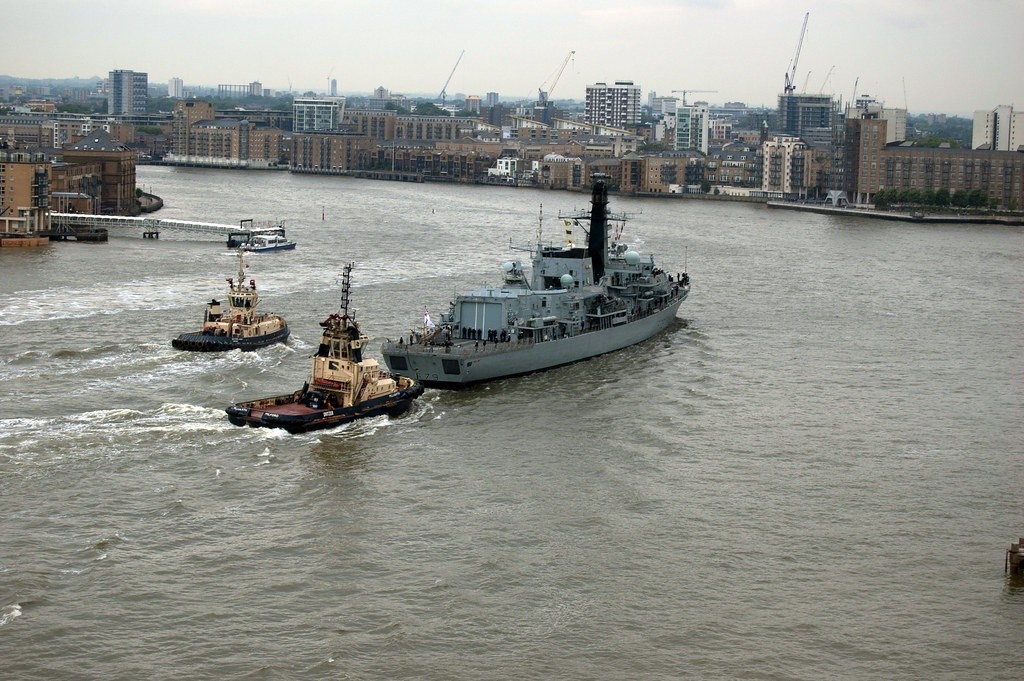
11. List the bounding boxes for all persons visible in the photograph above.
[400,336,403,344]
[462,326,507,342]
[409,334,413,346]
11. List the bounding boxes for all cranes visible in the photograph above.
[782,11,810,94]
[436,49,468,108]
[672,90,718,106]
[537,50,577,109]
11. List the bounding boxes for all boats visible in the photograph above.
[226,260,425,435]
[239,235,297,253]
[170,246,291,355]
[379,172,693,393]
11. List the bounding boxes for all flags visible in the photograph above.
[423,304,436,329]
